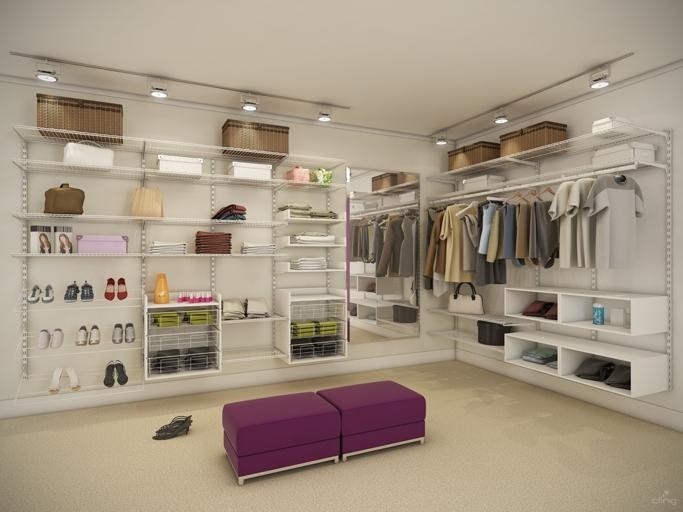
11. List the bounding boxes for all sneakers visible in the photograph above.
[38,329,50,349]
[52,328,64,348]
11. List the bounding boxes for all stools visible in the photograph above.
[317,379,426,463]
[221,391,341,486]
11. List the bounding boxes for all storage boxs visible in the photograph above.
[591,141,656,171]
[477,320,512,346]
[76,234,128,254]
[393,305,417,324]
[155,154,203,179]
[463,174,506,192]
[349,172,417,215]
[227,161,272,181]
[592,116,631,132]
[286,165,310,182]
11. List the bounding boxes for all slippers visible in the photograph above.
[48,367,81,395]
[103,360,128,387]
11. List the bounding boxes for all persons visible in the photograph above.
[58,233,72,253]
[39,233,51,253]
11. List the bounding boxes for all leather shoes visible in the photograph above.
[117,278,127,300]
[26,284,40,303]
[64,284,79,302]
[89,325,100,345]
[112,323,134,344]
[42,285,54,303]
[76,325,88,346]
[105,278,115,300]
[81,284,93,301]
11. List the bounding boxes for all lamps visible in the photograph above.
[33,57,64,84]
[145,76,336,124]
[435,68,611,146]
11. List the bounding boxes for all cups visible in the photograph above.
[592,304,604,324]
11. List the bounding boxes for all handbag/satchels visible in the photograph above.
[132,178,163,217]
[44,183,85,214]
[448,282,485,315]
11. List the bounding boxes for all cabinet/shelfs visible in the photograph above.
[349,181,420,339]
[11,124,351,419]
[427,125,670,400]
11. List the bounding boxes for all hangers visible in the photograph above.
[614,171,626,182]
[425,186,556,217]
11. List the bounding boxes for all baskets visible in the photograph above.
[499,120,567,162]
[448,141,500,170]
[371,172,419,196]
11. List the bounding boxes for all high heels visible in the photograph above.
[152,414,192,440]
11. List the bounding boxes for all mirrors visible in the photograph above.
[347,166,421,343]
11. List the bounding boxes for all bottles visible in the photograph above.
[154,273,168,303]
